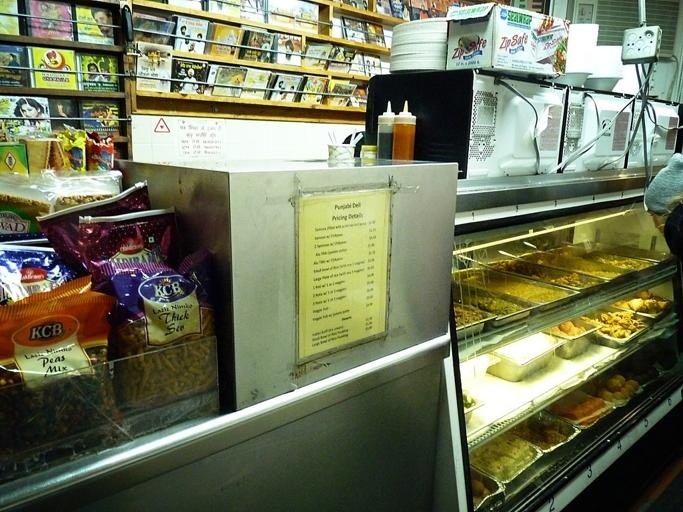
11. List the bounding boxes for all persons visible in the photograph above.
[643,152,683,282]
[6,1,114,134]
[176,26,295,103]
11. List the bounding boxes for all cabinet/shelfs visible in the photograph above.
[117,0,471,122]
[431,165,683,512]
[0,0,131,165]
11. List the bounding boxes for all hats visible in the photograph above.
[644,152,683,214]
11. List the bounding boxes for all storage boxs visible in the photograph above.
[447,3,572,79]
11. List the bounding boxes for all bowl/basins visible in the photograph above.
[585,76,622,91]
[552,71,592,86]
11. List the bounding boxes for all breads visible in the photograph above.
[554,321,587,337]
[512,413,573,449]
[585,308,646,338]
[592,374,639,401]
[472,481,489,505]
[552,391,612,425]
[470,433,534,480]
[612,289,670,314]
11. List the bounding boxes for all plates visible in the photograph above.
[389,17,449,73]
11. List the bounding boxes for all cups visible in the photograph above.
[327,144,357,162]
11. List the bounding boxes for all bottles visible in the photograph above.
[359,144,377,159]
[392,100,417,160]
[377,101,395,160]
